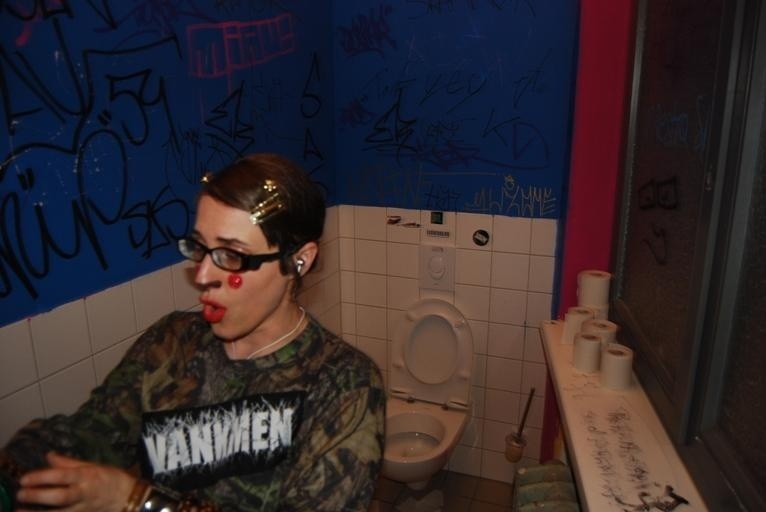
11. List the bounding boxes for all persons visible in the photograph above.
[0,151,387,511]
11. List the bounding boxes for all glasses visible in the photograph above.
[176,234,284,272]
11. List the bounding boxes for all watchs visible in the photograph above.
[138,481,182,511]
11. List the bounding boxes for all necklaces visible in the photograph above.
[230,302,305,362]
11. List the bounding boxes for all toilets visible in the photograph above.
[381,298,474,492]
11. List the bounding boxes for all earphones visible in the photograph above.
[294,256,306,279]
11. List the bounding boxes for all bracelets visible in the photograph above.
[121,478,152,511]
